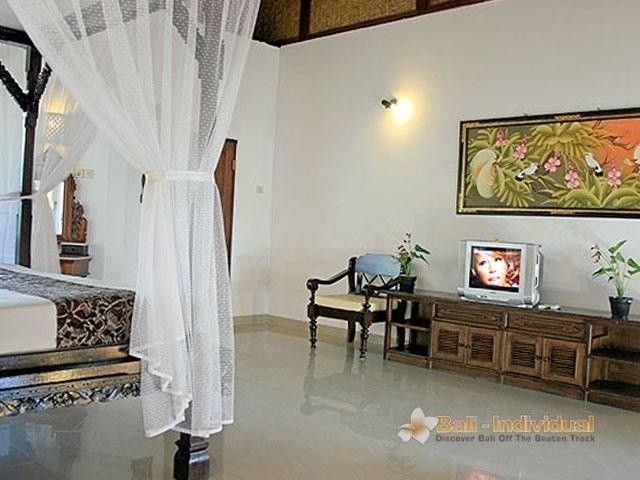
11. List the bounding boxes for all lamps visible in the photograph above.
[381,98,398,111]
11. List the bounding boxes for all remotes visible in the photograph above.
[538,304,550,309]
[554,304,561,310]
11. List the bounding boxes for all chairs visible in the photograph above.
[306,252,402,361]
[299,362,392,439]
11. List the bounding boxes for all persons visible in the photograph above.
[471,247,517,288]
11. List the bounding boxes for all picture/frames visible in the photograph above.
[456,106,640,219]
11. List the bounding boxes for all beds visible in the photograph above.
[0,0,226,480]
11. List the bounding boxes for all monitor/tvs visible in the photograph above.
[457,239,542,309]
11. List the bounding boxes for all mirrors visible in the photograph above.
[45,172,77,242]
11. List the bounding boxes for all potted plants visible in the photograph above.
[389,231,431,294]
[589,239,640,317]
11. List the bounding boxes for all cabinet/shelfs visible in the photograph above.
[59,242,93,278]
[378,288,640,412]
